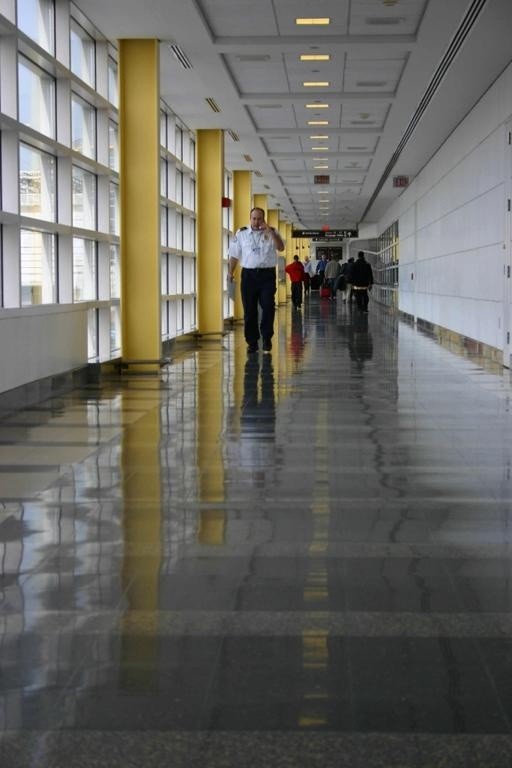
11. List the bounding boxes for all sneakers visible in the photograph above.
[260,339,273,352]
[245,345,260,355]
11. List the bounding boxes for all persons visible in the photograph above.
[285,250,374,310]
[227,207,285,352]
